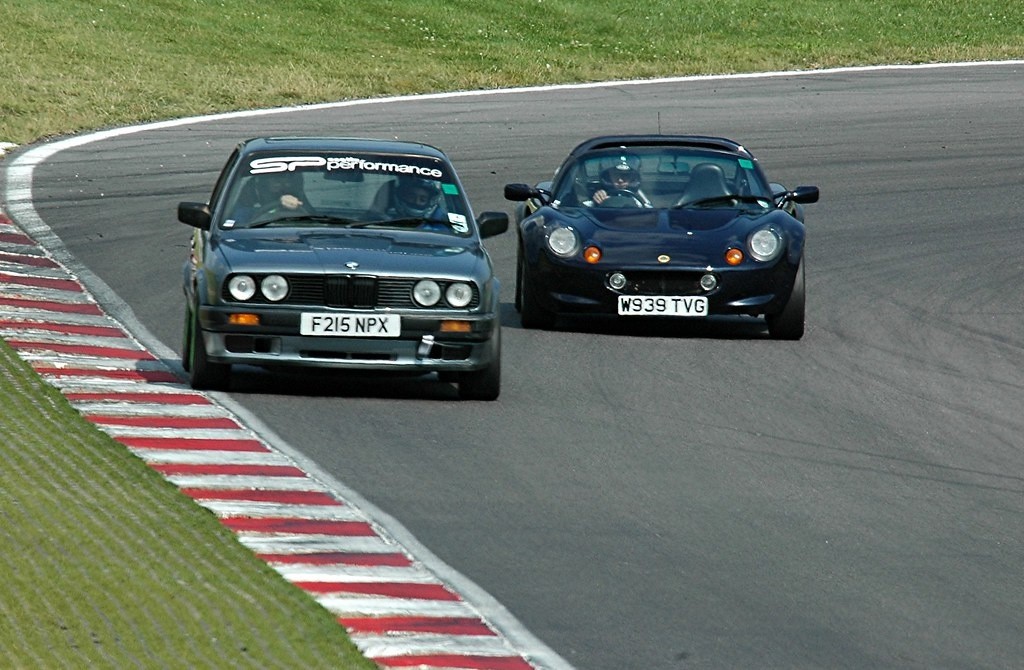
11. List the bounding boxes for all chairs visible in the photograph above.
[368,178,451,223]
[673,163,738,207]
[232,173,315,212]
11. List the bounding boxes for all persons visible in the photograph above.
[593,154,652,207]
[392,174,442,218]
[251,172,303,213]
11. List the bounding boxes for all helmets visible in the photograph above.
[598,152,642,197]
[392,173,443,219]
[255,171,303,206]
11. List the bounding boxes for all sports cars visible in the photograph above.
[501,133,821,341]
[175,135,510,402]
